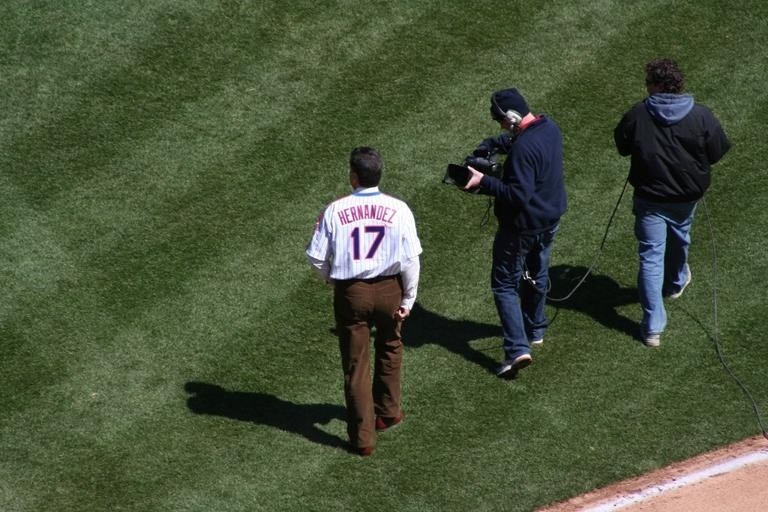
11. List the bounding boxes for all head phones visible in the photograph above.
[493,92,522,125]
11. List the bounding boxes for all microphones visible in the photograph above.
[508,124,514,143]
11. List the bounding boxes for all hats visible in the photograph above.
[489,88,530,122]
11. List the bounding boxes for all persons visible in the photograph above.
[300,146,424,458]
[612,59,734,348]
[454,89,569,380]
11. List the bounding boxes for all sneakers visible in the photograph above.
[665,263,691,299]
[357,412,403,456]
[639,320,660,346]
[495,338,543,379]
